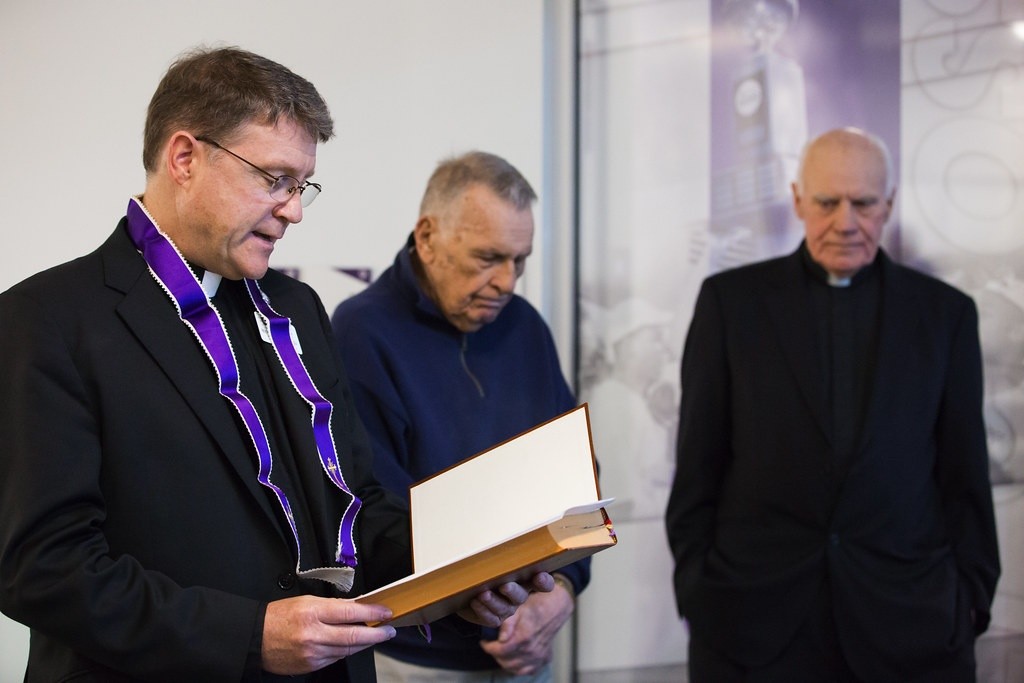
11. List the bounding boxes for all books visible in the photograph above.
[352,404,617,628]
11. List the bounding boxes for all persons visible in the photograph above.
[328,150,592,683]
[0,50,555,683]
[664,126,1002,683]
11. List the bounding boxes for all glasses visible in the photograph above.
[195,136,322,207]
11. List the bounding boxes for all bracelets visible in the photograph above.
[554,576,571,592]
